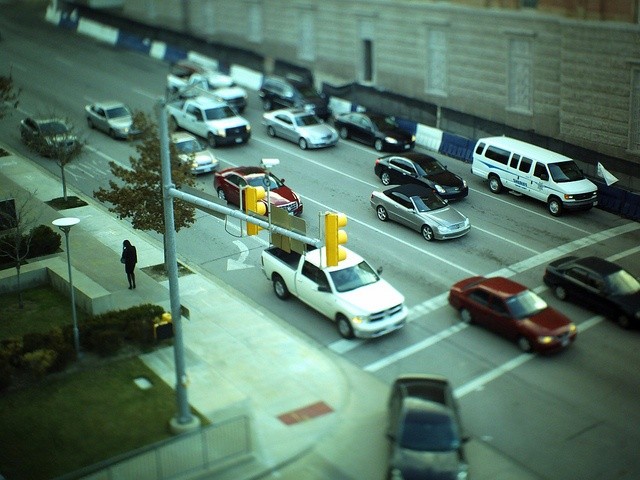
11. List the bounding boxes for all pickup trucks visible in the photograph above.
[168,59,250,114]
[384,376,472,479]
[261,241,408,339]
[166,97,251,145]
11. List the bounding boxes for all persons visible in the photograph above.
[120,240,137,290]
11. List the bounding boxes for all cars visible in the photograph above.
[84,100,143,138]
[335,111,415,152]
[262,108,338,149]
[214,166,303,218]
[543,256,640,332]
[171,131,219,175]
[20,114,78,156]
[375,152,468,201]
[370,184,471,241]
[449,276,576,354]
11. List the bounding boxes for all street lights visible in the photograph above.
[52,216,85,362]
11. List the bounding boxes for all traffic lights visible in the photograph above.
[325,211,349,268]
[243,184,268,237]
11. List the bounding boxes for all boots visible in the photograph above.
[128,277,132,289]
[132,275,136,288]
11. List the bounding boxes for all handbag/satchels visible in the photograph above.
[120,257,126,263]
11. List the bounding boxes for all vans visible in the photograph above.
[471,135,599,217]
[258,76,332,121]
